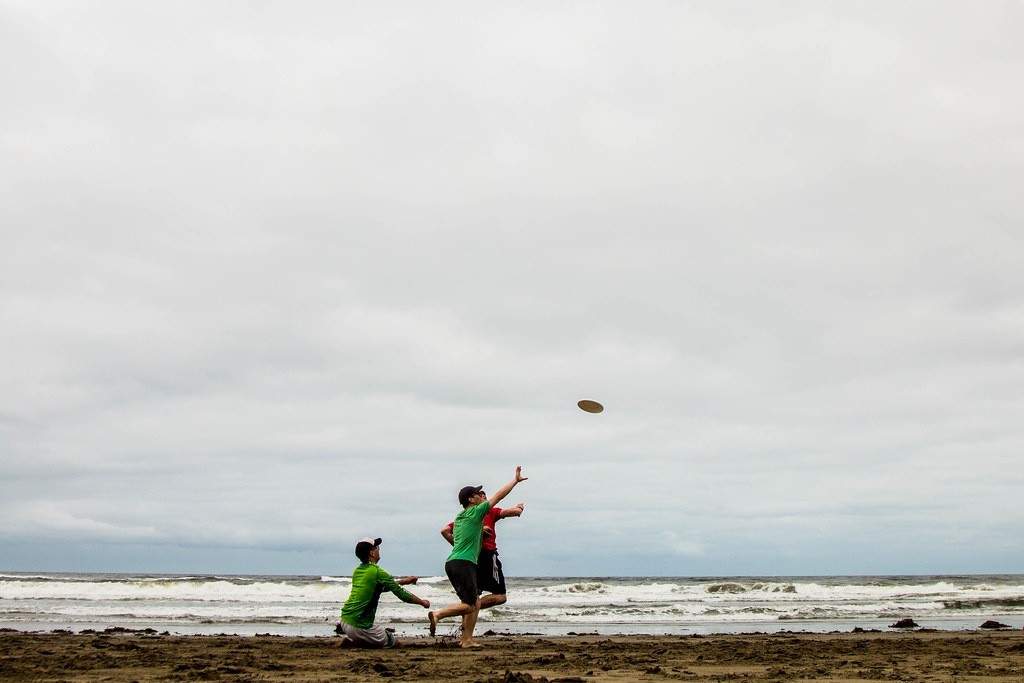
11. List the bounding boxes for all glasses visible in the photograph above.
[475,492,482,497]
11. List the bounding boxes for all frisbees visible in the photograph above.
[576,399,604,414]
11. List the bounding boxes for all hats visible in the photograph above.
[354,537,382,558]
[458,485,483,505]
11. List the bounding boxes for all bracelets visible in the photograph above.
[519,507,524,511]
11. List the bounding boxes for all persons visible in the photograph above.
[333,538,430,650]
[428,466,528,648]
